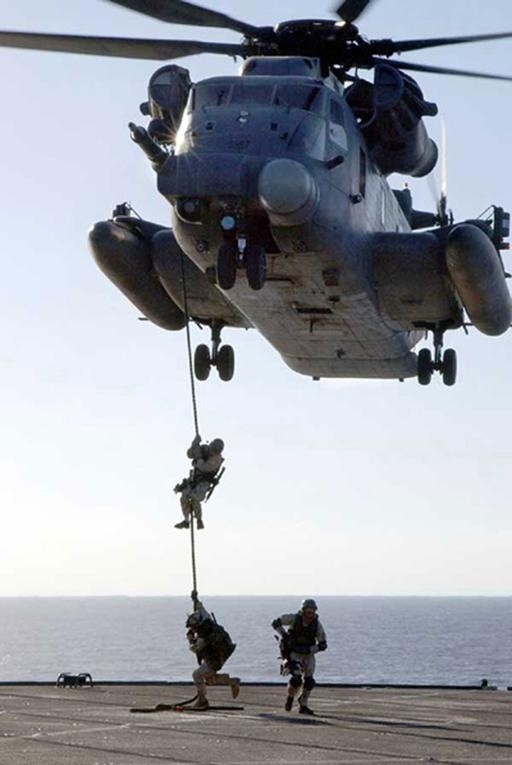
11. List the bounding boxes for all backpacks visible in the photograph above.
[199,620,237,658]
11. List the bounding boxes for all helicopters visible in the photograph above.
[0,1,510,386]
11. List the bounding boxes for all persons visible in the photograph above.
[270,597,328,714]
[173,432,226,529]
[181,589,240,710]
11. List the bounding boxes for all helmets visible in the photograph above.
[301,598,318,611]
[208,438,225,453]
[185,612,205,628]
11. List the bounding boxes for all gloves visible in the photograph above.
[191,434,204,459]
[186,629,194,641]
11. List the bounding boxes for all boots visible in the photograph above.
[195,518,205,530]
[284,696,294,712]
[174,520,190,529]
[192,697,210,712]
[298,706,314,715]
[229,677,241,699]
